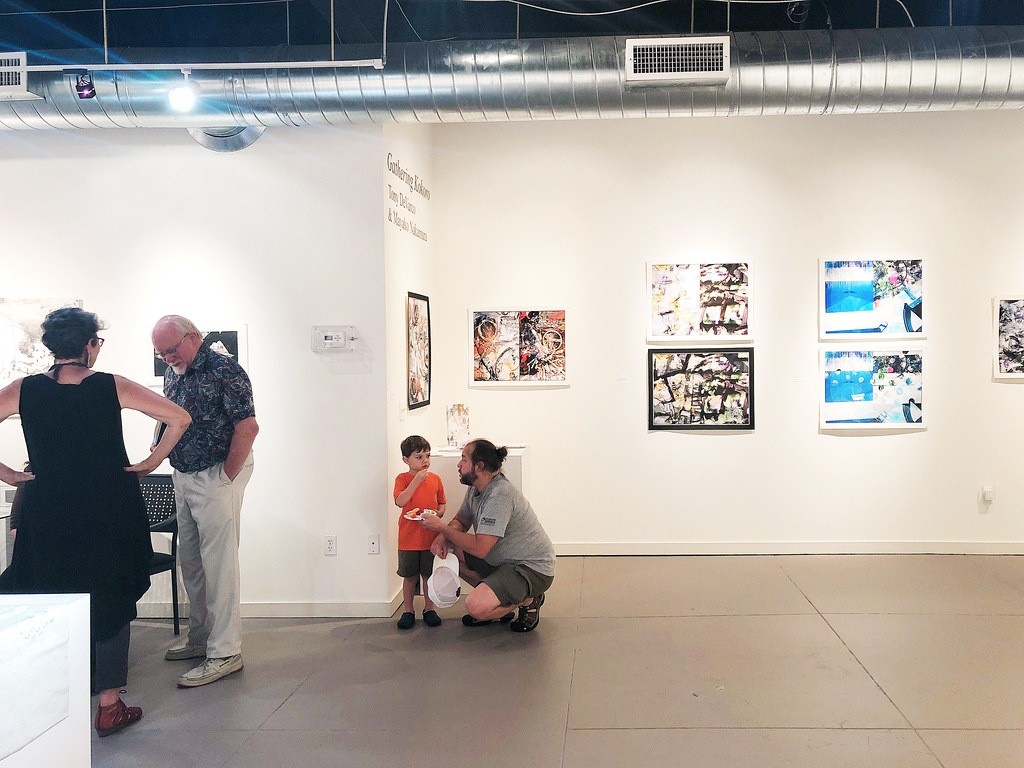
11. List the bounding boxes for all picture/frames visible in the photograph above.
[406,291,431,409]
[818,344,926,429]
[648,348,755,431]
[818,256,925,339]
[646,261,754,341]
[993,295,1024,379]
[145,324,247,387]
[467,307,570,386]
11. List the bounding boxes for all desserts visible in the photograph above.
[406,508,434,519]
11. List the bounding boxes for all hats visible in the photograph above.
[427,553,461,608]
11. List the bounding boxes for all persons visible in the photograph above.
[0,303,193,736]
[430,440,556,633]
[152,316,261,688]
[394,436,447,628]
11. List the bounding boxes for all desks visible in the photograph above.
[420,448,523,594]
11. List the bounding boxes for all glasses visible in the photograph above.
[154,333,188,359]
[87,336,104,347]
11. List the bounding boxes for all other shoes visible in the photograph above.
[397,611,415,629]
[94,698,143,737]
[423,609,441,626]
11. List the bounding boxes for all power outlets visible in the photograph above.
[324,535,337,555]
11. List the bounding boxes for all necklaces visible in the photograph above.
[48,361,87,380]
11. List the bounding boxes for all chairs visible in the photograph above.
[139,474,180,636]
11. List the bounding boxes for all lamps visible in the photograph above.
[0,0,390,113]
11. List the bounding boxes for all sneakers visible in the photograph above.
[165,642,206,660]
[510,592,545,632]
[462,612,515,626]
[177,654,243,686]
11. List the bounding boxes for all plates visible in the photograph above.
[404,510,437,520]
